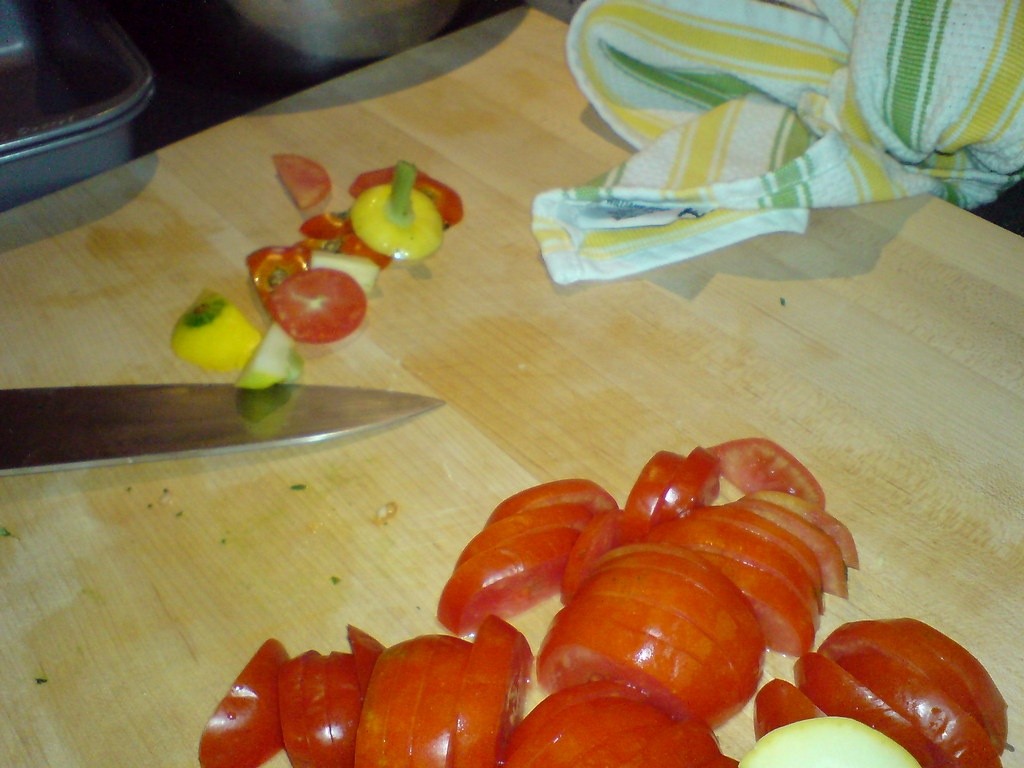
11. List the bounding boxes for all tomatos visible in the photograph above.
[245,152,463,344]
[197,440,1007,768]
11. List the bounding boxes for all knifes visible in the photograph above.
[0,385,445,479]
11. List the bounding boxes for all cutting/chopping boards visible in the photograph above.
[0,9,1024,768]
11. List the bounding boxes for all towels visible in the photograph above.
[532,0,1023,285]
